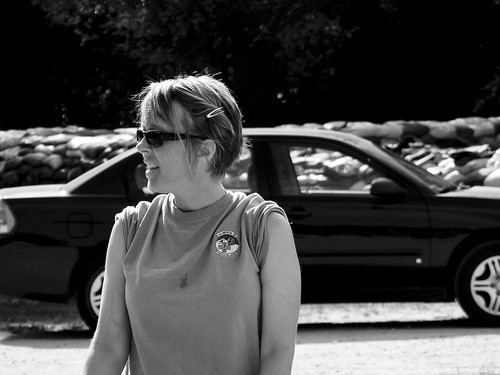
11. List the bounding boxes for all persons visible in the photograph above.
[82,76,301,375]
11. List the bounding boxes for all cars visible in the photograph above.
[1,128,500,334]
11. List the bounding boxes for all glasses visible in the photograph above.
[137,130,207,147]
